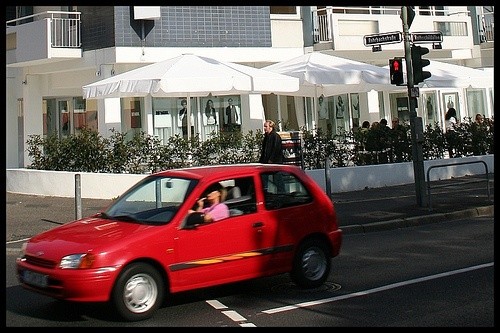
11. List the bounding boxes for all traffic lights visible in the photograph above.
[411,44,432,86]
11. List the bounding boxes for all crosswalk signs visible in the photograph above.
[389,58,404,85]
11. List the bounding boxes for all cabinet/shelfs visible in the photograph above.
[273,139,304,183]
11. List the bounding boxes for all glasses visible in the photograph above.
[392,120,398,123]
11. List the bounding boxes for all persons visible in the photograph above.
[205,99,217,141]
[427,97,432,123]
[357,118,389,164]
[226,98,238,125]
[336,96,346,135]
[316,97,328,135]
[391,118,408,160]
[447,96,453,109]
[258,120,284,193]
[194,182,230,223]
[180,100,195,142]
[445,108,460,158]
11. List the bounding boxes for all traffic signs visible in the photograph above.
[409,31,444,49]
[398,6,416,30]
[363,31,403,52]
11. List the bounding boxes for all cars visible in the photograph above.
[16,162,344,321]
